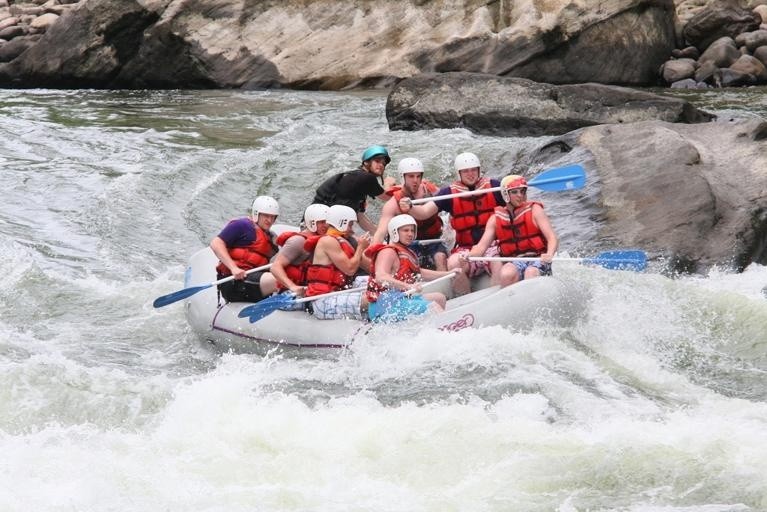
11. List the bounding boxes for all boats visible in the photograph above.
[182,224,566,358]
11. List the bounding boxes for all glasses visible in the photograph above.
[509,189,526,196]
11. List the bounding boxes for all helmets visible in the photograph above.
[325,204,359,232]
[361,145,393,166]
[499,175,529,204]
[452,151,482,183]
[386,215,418,243]
[305,204,329,233]
[399,157,425,188]
[252,194,281,224]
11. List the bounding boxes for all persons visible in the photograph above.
[304,205,371,320]
[364,214,463,327]
[270,204,330,311]
[399,152,507,298]
[209,196,279,302]
[370,158,448,272]
[313,145,392,237]
[461,174,558,289]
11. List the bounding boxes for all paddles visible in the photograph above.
[411,165,586,205]
[250,286,307,324]
[468,251,647,271]
[376,272,457,317]
[153,262,273,308]
[238,286,367,318]
